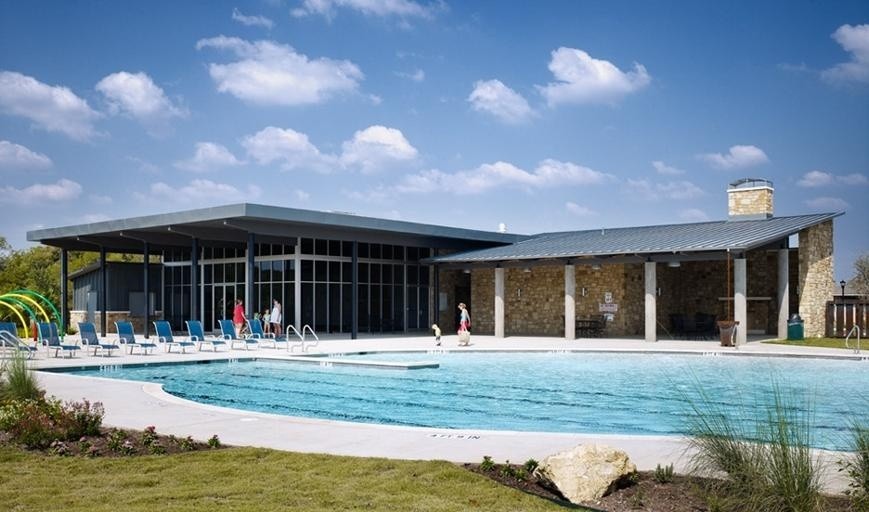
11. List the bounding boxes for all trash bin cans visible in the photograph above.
[787,313,804,340]
[716,320,739,347]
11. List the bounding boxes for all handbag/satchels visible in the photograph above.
[458,331,470,343]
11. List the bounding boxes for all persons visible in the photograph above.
[456,302,471,346]
[232,299,247,340]
[270,297,282,338]
[432,324,445,346]
[261,309,272,337]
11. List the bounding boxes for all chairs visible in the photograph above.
[0,319,289,361]
[561,312,608,338]
